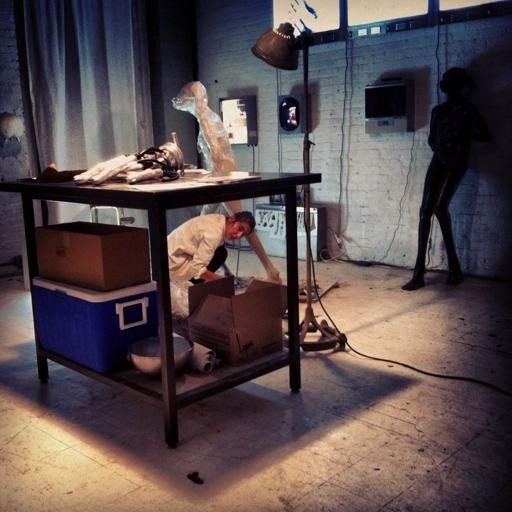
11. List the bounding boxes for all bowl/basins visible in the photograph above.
[128,336,194,378]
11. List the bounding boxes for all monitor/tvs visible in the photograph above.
[272,0,512,47]
[219,96,258,145]
[363,81,415,134]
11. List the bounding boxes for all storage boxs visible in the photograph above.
[33,219,158,375]
[188,277,283,367]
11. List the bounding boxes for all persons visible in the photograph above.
[167,210,256,284]
[401,66,493,291]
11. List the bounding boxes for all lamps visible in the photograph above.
[250,21,347,352]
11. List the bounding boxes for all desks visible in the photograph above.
[0,167,322,448]
